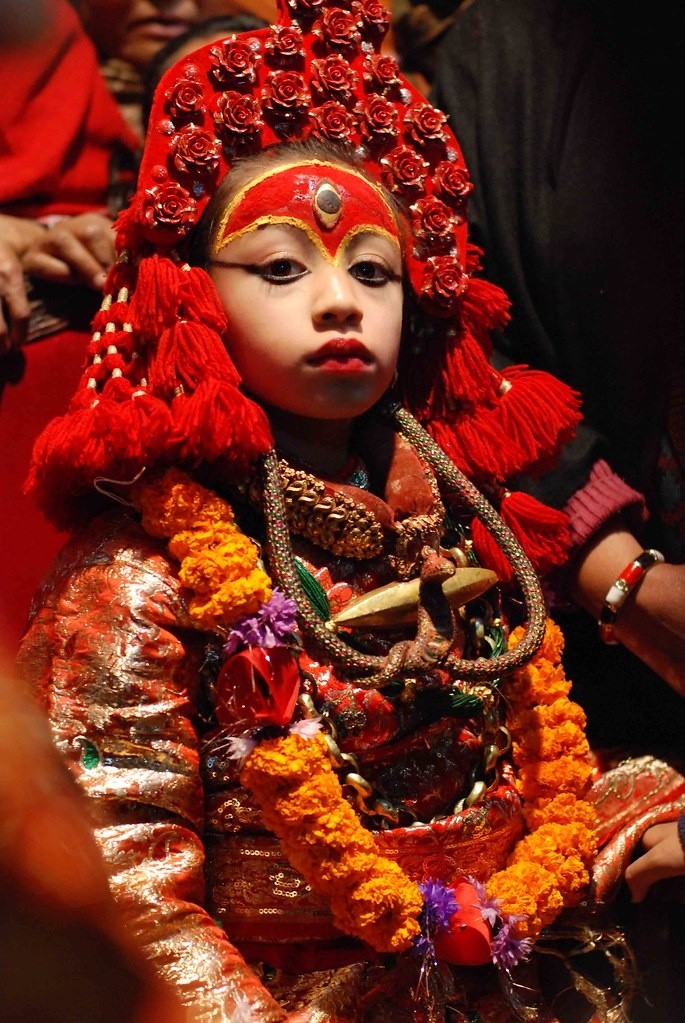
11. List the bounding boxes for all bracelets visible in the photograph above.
[600,548,665,645]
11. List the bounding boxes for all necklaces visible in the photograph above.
[265,407,545,682]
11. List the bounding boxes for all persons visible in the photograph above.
[1,0,685,1021]
[15,147,685,1023]
